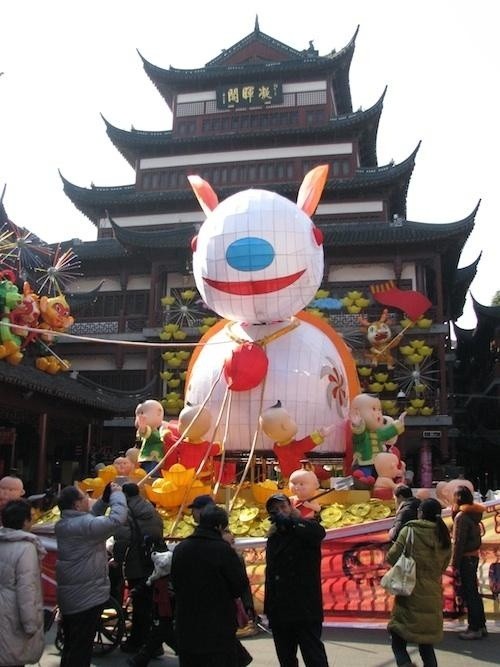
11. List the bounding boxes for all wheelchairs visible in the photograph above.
[54,537,136,658]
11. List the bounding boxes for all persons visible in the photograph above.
[115,397,224,490]
[54,481,128,666]
[0,471,26,508]
[256,390,408,521]
[168,504,257,666]
[187,494,214,525]
[451,485,488,640]
[263,493,328,666]
[382,483,421,550]
[385,499,453,667]
[113,483,164,659]
[1,498,46,666]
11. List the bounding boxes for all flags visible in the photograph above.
[369,278,434,323]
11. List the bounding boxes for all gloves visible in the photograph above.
[269,511,292,529]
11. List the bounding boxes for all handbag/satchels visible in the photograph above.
[380,552,416,595]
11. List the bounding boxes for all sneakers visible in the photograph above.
[458,626,487,639]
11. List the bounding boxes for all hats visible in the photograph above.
[187,494,215,509]
[266,492,290,508]
